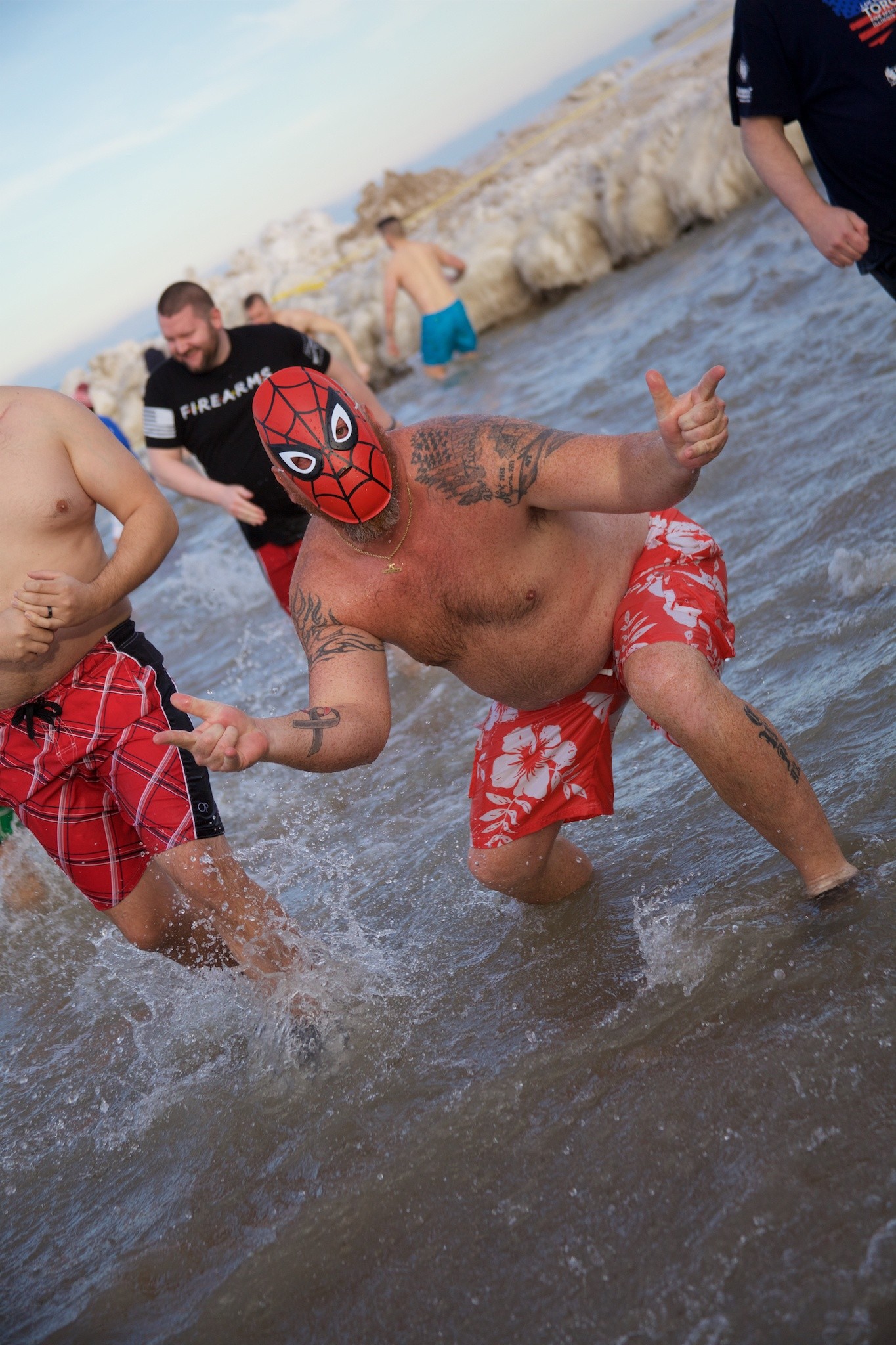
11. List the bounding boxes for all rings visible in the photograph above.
[48,606,52,618]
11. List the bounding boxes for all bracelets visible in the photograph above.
[385,417,396,431]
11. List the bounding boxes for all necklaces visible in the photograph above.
[334,482,412,573]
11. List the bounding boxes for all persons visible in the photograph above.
[728,0,896,301]
[144,347,166,373]
[141,282,404,614]
[153,366,857,900]
[376,216,478,378]
[0,385,302,995]
[241,294,371,380]
[74,383,130,452]
